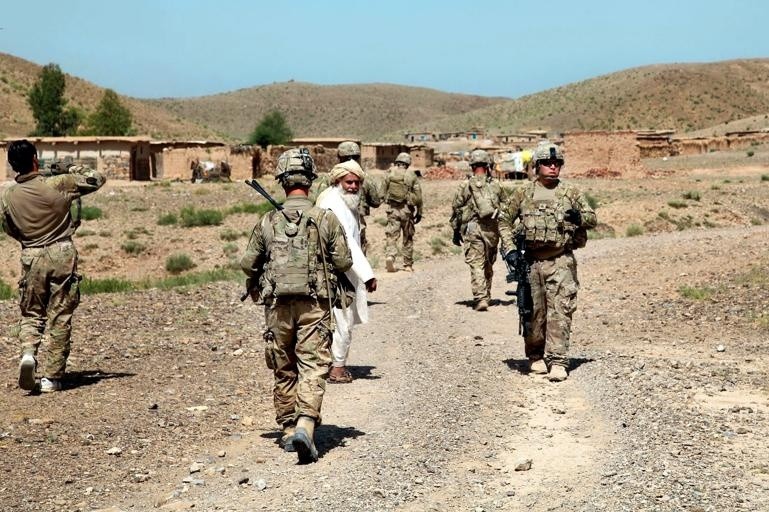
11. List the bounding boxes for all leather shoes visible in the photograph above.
[328,366,353,382]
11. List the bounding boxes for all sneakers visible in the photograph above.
[548,364,569,381]
[404,265,413,272]
[18,354,36,390]
[473,299,489,311]
[386,255,396,272]
[528,358,548,374]
[39,378,61,393]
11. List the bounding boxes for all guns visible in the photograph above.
[505,236,533,337]
[245,179,354,294]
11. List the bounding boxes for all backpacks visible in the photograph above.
[389,175,408,200]
[470,179,495,218]
[259,207,324,298]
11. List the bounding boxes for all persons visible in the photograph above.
[449,149,509,311]
[496,140,597,383]
[380,152,425,276]
[238,148,353,466]
[219,159,234,179]
[315,139,381,249]
[0,134,108,395]
[312,158,378,385]
[494,146,537,183]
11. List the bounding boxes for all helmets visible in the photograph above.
[469,149,491,166]
[395,152,411,165]
[274,147,318,180]
[336,141,361,157]
[530,140,564,167]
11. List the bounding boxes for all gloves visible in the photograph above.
[506,250,521,268]
[564,208,581,226]
[413,213,422,224]
[50,161,72,174]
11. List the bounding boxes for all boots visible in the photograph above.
[283,416,319,464]
